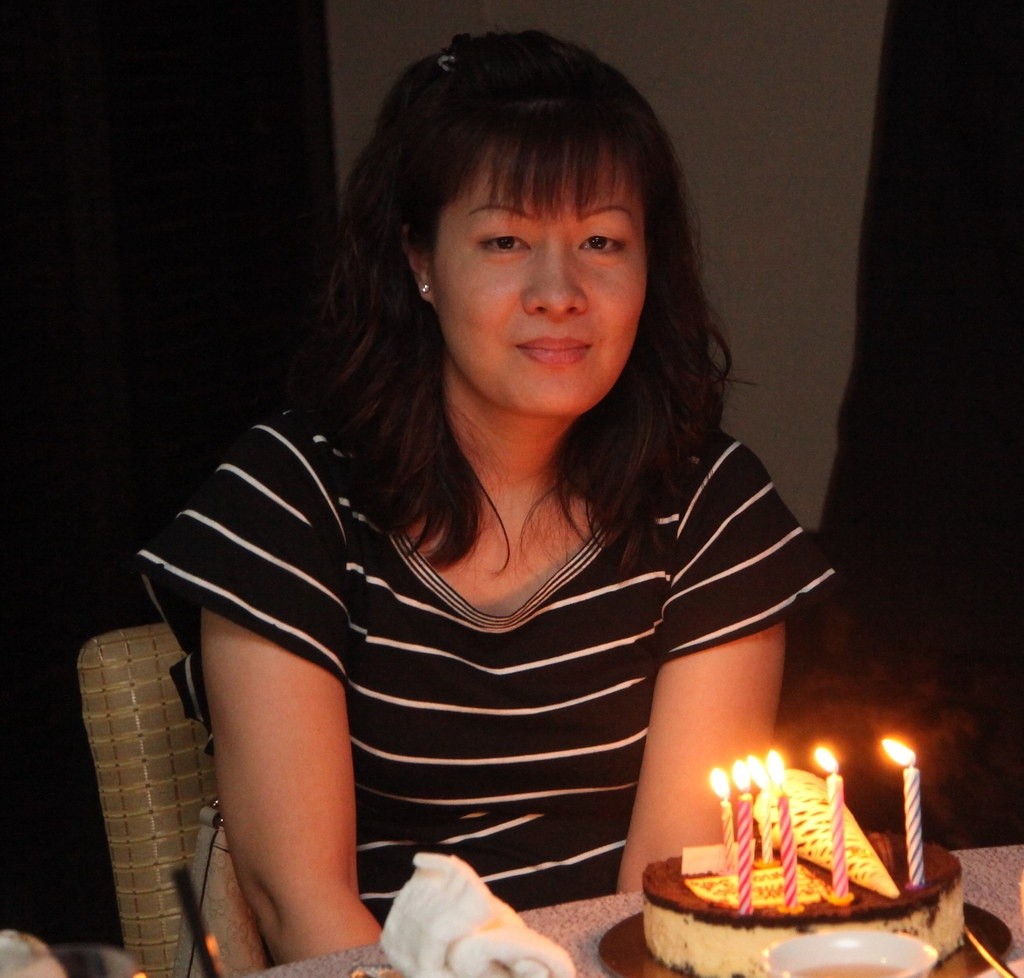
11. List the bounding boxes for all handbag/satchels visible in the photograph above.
[170,804,273,978]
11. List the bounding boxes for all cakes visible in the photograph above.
[642,828,964,978]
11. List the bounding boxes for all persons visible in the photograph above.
[140,30,837,968]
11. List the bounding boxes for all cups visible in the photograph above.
[760,929,938,978]
[0,942,144,978]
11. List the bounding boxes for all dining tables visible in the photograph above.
[515,845,1023,978]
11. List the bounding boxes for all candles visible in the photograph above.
[767,750,798,908]
[882,738,926,886]
[714,755,772,916]
[812,748,849,901]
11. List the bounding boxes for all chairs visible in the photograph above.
[77,620,228,976]
